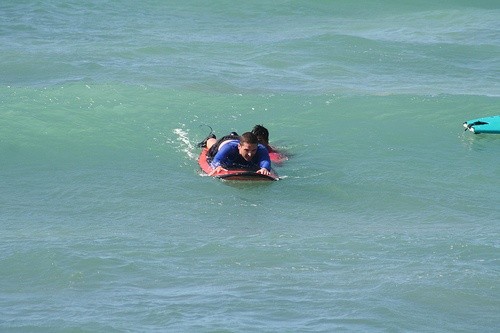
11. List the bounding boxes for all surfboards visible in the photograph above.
[263,147,289,163]
[462,114,500,133]
[197,148,280,181]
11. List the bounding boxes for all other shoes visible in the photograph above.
[196,134,216,148]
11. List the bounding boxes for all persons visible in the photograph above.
[196,131,271,175]
[250,125,277,153]
